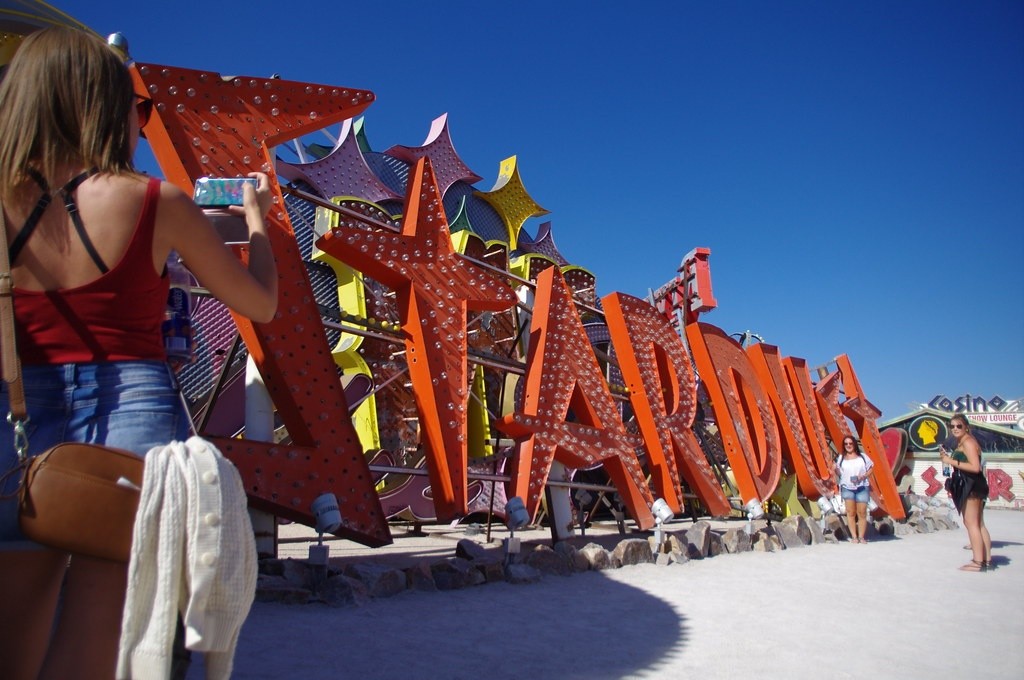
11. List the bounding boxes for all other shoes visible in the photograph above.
[964,545,973,549]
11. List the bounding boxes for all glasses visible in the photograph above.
[950,425,962,429]
[843,443,854,446]
[135,93,153,129]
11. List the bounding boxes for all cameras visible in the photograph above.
[943,466,950,476]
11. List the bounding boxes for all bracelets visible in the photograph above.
[955,460,960,470]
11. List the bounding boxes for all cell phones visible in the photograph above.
[192,177,258,207]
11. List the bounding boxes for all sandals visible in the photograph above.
[858,537,867,544]
[986,561,994,571]
[850,537,859,544]
[958,559,987,572]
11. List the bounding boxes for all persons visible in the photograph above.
[833,435,874,544]
[940,414,992,572]
[0,26,278,680]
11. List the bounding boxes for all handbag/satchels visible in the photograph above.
[17,441,144,564]
